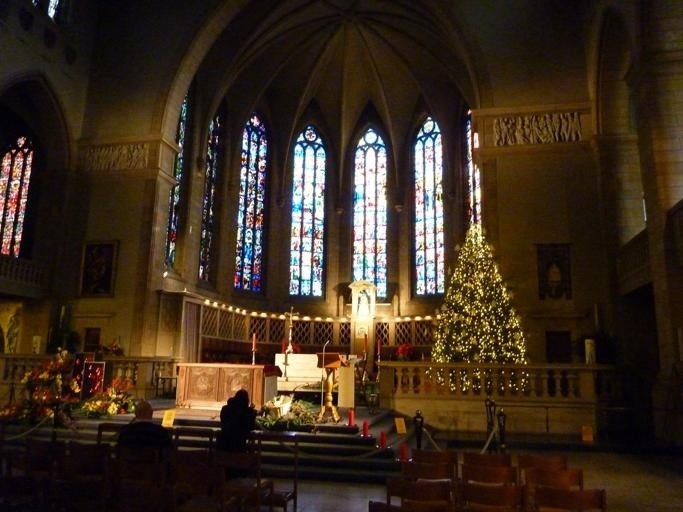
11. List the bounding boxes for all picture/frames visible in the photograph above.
[76,239,120,298]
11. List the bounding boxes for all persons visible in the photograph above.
[80,144,149,170]
[215,388,258,479]
[115,400,174,465]
[493,110,583,147]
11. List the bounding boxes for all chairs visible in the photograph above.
[369,447,608,512]
[0,423,299,512]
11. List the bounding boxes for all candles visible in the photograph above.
[348,410,355,426]
[380,431,387,448]
[363,419,369,438]
[377,339,381,353]
[400,442,407,463]
[364,334,367,351]
[253,333,256,350]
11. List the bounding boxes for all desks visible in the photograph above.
[175,363,282,413]
